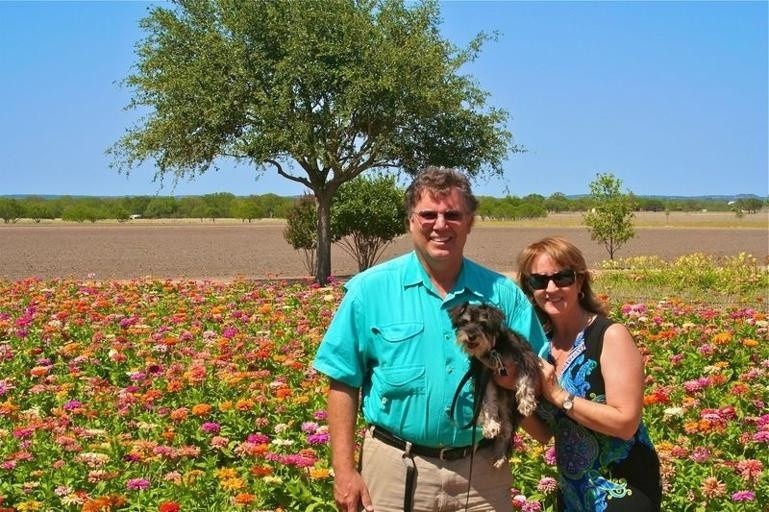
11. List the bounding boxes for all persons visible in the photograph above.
[514,235,662,512]
[310,168,555,512]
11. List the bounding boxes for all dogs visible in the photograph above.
[447,298,543,470]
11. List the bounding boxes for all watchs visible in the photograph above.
[560,393,576,414]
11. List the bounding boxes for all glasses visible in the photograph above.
[528,269,576,289]
[413,210,462,220]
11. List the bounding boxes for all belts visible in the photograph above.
[367,424,495,460]
